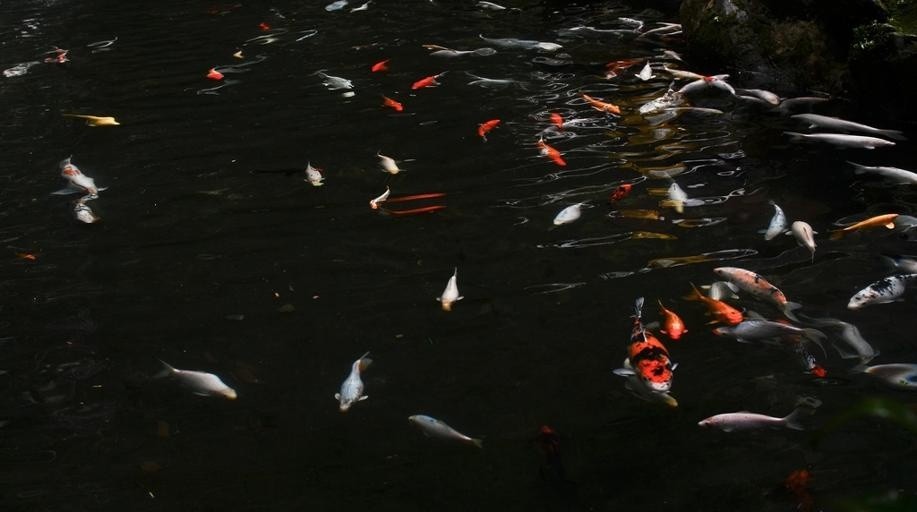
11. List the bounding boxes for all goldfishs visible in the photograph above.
[433,268,464,313]
[616,264,917,433]
[336,352,372,415]
[307,3,917,252]
